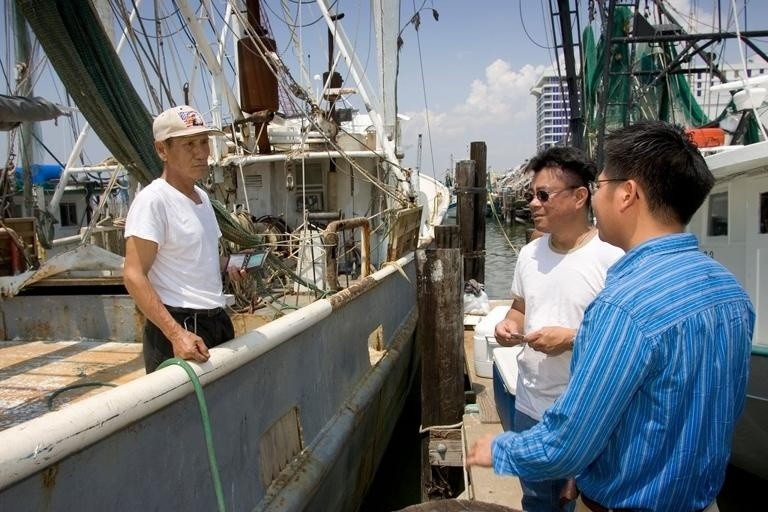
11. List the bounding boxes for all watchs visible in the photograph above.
[568,337,576,352]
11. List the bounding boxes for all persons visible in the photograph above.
[122,104,257,374]
[494,144,626,512]
[464,118,754,511]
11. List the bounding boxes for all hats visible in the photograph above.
[154,105,224,141]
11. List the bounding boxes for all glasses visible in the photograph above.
[522,188,571,202]
[589,177,640,199]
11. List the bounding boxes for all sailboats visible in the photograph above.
[1,0,452,512]
[525,1,768,481]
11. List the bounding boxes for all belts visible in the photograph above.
[580,493,628,512]
[165,307,222,318]
